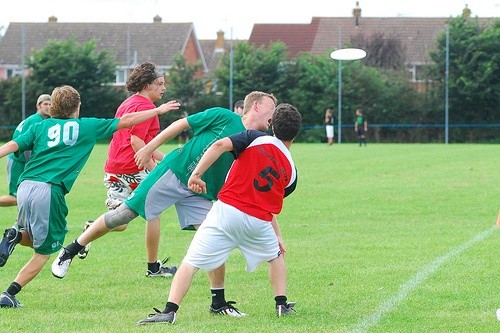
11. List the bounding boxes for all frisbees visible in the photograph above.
[329,47,367,61]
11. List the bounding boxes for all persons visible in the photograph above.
[325,107,335,146]
[234,100,247,119]
[182,110,190,144]
[353,108,369,146]
[0,85,180,309]
[0,95,52,207]
[137,104,302,327]
[52,91,279,319]
[79,60,179,278]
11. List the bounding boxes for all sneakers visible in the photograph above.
[0,227,19,267]
[51,248,72,279]
[1,291,26,307]
[136,307,176,325]
[208,300,249,318]
[275,302,298,318]
[77,220,96,259]
[145,262,177,277]
